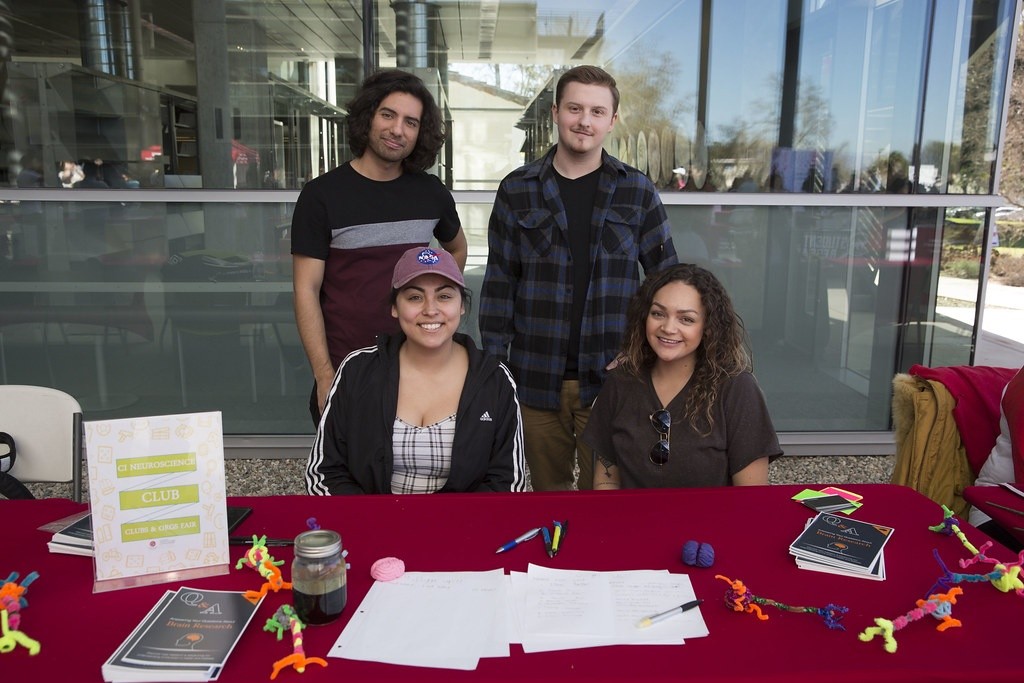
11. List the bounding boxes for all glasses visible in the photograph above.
[648,407,671,466]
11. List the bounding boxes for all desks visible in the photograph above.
[0,483,1024,683]
[962,484,1024,540]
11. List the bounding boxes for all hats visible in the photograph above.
[390,245,465,291]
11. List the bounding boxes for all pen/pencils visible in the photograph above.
[552,522,562,554]
[555,520,568,556]
[644,599,704,626]
[496,527,541,553]
[230,537,294,546]
[542,527,553,559]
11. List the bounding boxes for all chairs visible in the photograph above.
[0,385,83,501]
[890,364,1021,522]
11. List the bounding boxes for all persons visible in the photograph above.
[576,259,785,490]
[302,244,529,497]
[475,63,680,495]
[285,65,474,437]
[13,154,143,225]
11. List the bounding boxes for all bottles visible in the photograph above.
[291,529,348,625]
